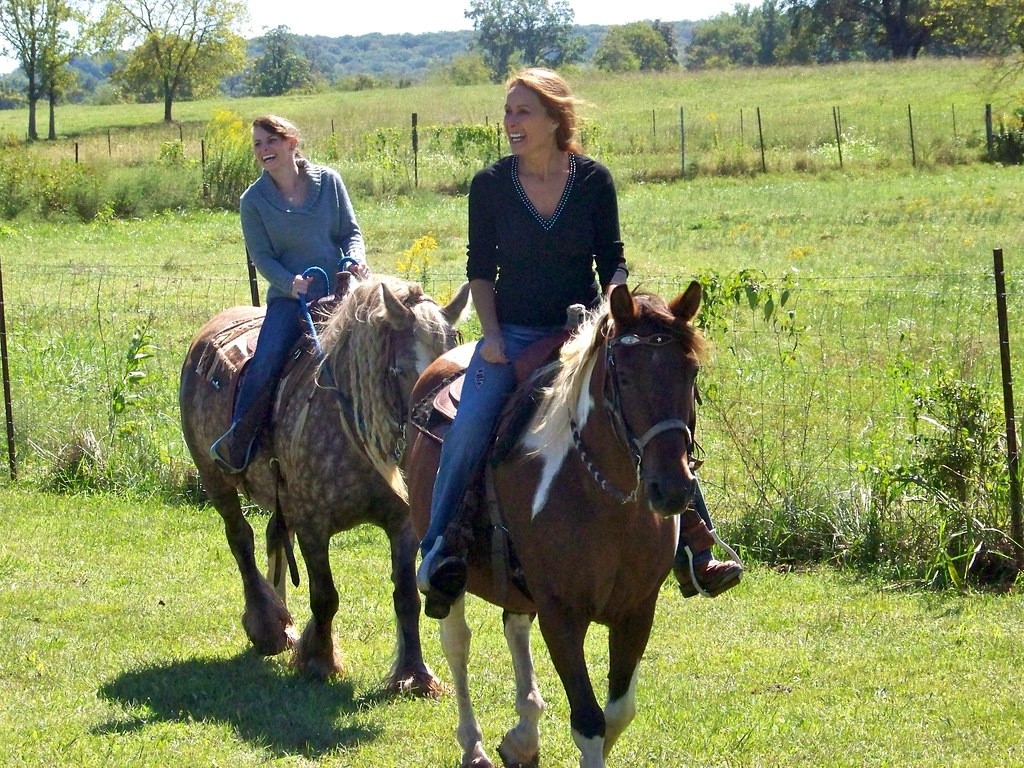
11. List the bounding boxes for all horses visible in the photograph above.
[410,279,705,766]
[179,279,471,702]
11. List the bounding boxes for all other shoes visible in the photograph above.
[229,418,262,470]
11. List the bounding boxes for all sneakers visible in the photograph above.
[673,558,742,598]
[425,555,467,619]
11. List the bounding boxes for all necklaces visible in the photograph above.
[286,171,301,202]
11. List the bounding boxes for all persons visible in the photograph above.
[419,69,742,621]
[220,114,371,468]
